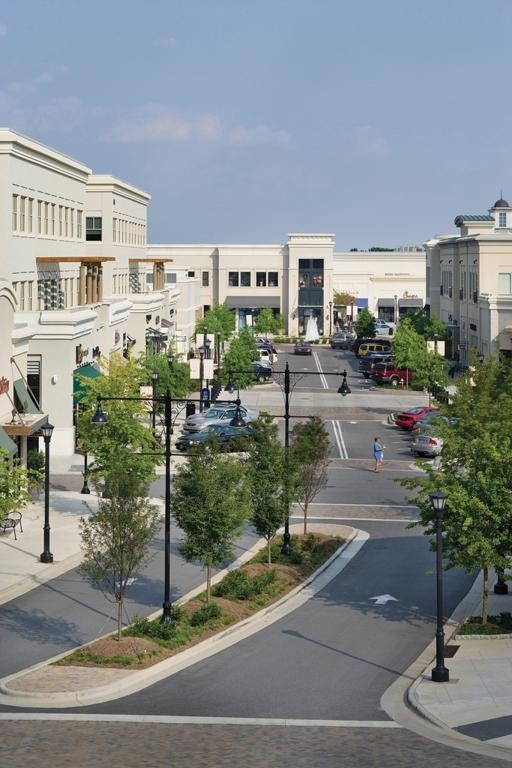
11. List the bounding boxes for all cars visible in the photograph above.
[249,336,279,382]
[329,317,414,386]
[174,403,262,452]
[394,406,457,457]
[294,342,313,355]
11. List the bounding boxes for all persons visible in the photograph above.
[373,436,386,473]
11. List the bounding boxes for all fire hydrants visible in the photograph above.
[398,378,405,390]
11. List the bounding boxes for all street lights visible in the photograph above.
[90,388,248,628]
[426,487,454,681]
[151,372,160,440]
[394,294,398,323]
[39,416,55,564]
[166,351,175,367]
[349,296,355,321]
[227,361,352,557]
[327,301,333,337]
[198,325,221,413]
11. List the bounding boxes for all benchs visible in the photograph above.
[0,512,23,541]
